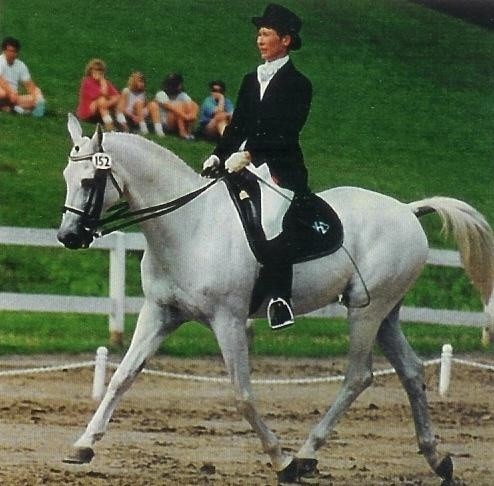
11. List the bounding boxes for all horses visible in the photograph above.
[55,111,493,482]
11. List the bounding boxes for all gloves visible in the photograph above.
[203,155,220,169]
[225,152,249,174]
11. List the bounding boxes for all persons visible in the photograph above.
[0,36,48,118]
[154,72,200,143]
[204,3,312,333]
[74,57,119,131]
[116,72,165,138]
[199,78,233,143]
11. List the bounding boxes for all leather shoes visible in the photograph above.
[270,302,291,325]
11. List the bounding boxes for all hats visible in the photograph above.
[252,3,301,50]
